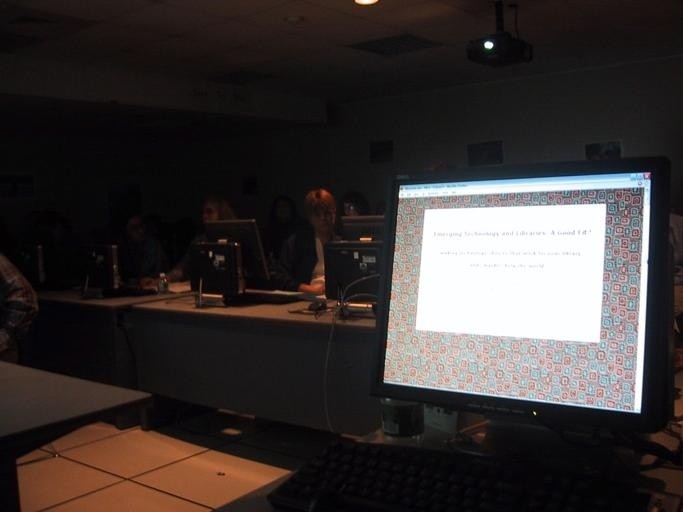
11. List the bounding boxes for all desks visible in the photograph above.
[0,357,153,512]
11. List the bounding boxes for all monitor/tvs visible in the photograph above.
[77,244,112,298]
[366,156,671,458]
[336,215,385,241]
[203,219,271,306]
[322,240,385,319]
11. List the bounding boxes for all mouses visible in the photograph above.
[308,302,327,310]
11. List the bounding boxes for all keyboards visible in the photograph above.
[225,293,305,304]
[102,289,158,298]
[266,437,652,512]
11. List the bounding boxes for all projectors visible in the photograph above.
[468,31,532,68]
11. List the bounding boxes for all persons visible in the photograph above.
[264,190,371,256]
[265,188,342,295]
[0,252,38,364]
[115,210,163,294]
[139,198,243,290]
[667,214,682,340]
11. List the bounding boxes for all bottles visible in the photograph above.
[157,272,168,296]
